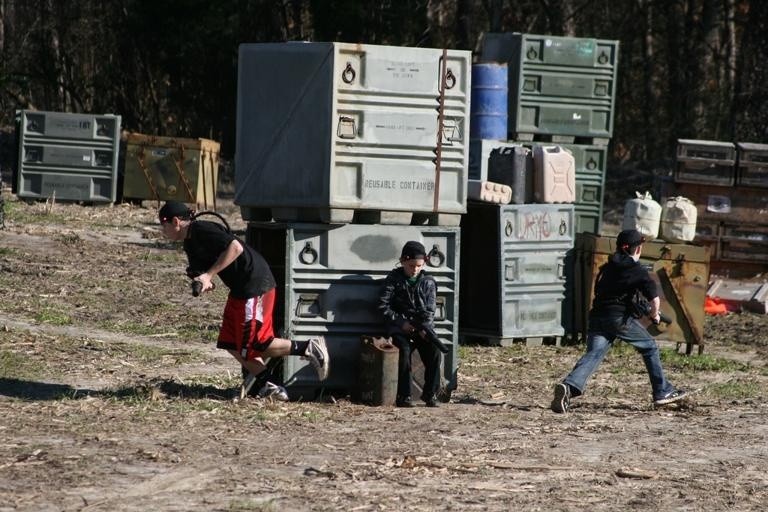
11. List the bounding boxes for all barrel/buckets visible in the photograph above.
[662,194,699,241]
[534,142,578,205]
[489,145,531,203]
[472,61,509,140]
[623,190,662,239]
[361,334,401,406]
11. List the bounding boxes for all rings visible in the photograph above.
[409,328,412,331]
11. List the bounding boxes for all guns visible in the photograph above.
[391,294,449,354]
[186,267,215,297]
[624,291,671,327]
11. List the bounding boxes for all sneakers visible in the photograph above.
[652,389,685,406]
[551,384,571,414]
[255,382,288,401]
[300,336,329,381]
[420,396,440,407]
[396,396,417,408]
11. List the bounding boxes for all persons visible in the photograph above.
[159,201,330,402]
[552,230,686,414]
[375,241,443,408]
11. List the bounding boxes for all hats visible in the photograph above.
[617,229,646,249]
[400,241,427,260]
[160,200,192,220]
